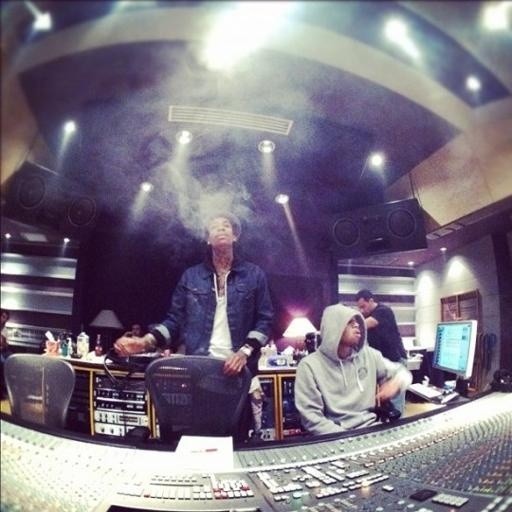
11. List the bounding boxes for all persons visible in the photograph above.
[124,322,157,352]
[0,308,14,399]
[293,303,414,435]
[355,288,409,412]
[113,210,278,377]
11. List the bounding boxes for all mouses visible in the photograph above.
[432,396,444,400]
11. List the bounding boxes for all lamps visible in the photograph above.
[283,317,319,338]
[88,310,125,351]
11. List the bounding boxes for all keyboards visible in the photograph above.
[410,382,441,399]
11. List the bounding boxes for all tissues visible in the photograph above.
[45,331,59,354]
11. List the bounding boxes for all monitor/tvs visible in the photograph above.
[432,319,478,378]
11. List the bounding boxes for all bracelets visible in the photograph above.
[239,346,252,357]
[148,332,158,345]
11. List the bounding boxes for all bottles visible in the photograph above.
[76,332,90,359]
[95,334,103,356]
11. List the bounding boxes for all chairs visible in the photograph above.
[4,354,77,428]
[123,355,253,447]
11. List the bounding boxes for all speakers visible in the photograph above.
[324,197,427,257]
[3,158,103,235]
